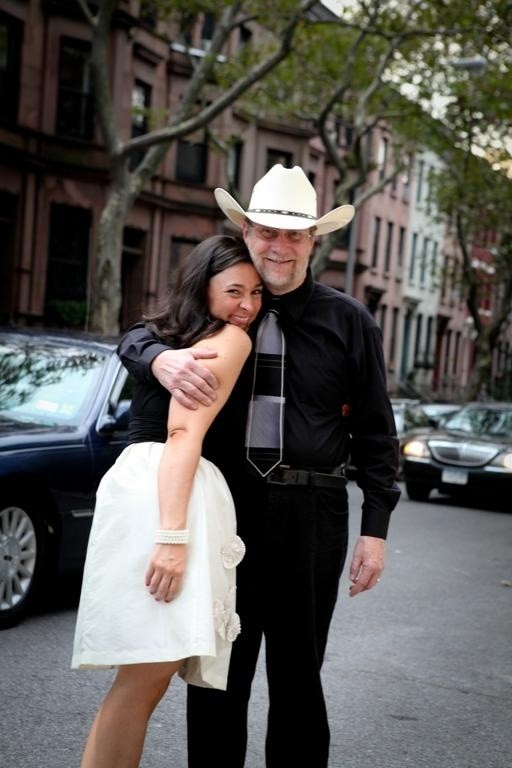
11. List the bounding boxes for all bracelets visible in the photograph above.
[153,529,191,546]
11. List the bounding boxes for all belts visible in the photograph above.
[266,464,350,489]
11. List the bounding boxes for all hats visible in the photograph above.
[213,163,356,235]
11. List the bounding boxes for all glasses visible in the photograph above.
[248,224,312,246]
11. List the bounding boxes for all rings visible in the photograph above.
[377,579,379,582]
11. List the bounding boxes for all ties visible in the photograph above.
[245,307,286,478]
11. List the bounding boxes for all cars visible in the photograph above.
[1,327,131,632]
[390,401,511,507]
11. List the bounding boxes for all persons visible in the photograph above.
[114,159,404,766]
[67,231,275,767]
